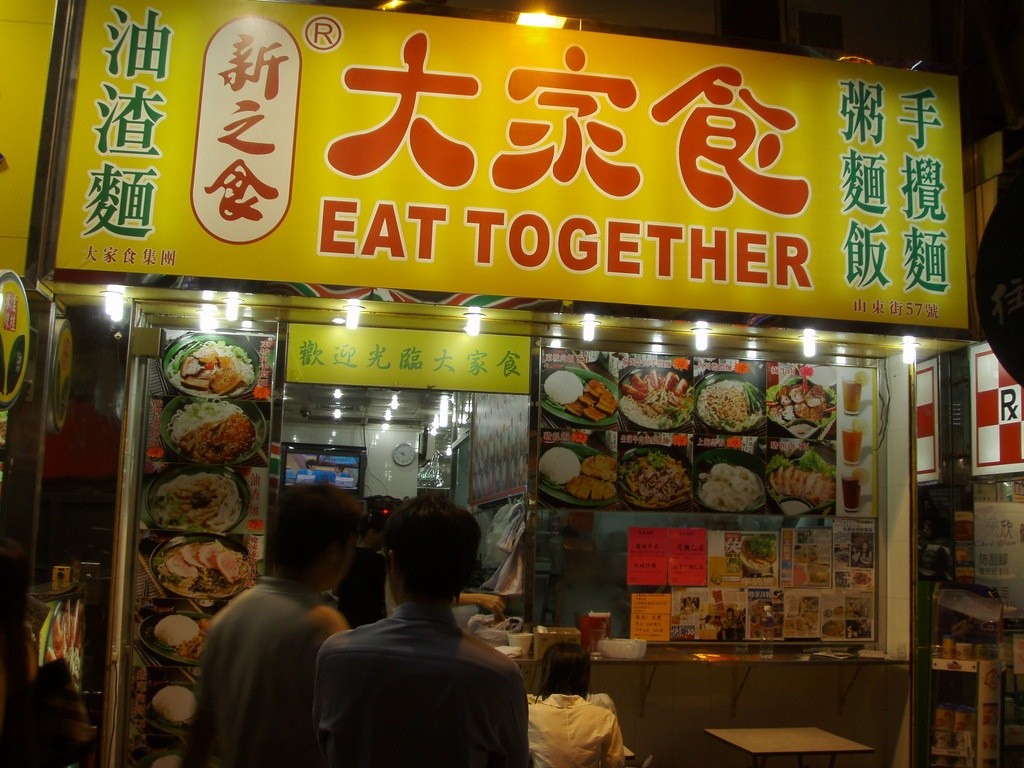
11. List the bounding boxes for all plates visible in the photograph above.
[539,441,616,506]
[540,366,616,425]
[767,376,836,440]
[134,750,221,768]
[138,681,200,735]
[138,611,214,665]
[768,458,836,514]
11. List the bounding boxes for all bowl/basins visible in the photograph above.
[159,393,267,465]
[618,367,693,431]
[618,446,693,508]
[160,331,263,399]
[149,532,258,599]
[145,464,251,532]
[694,372,765,435]
[693,449,767,511]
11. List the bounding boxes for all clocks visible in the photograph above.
[393,443,415,465]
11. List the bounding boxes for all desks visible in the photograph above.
[705,727,875,768]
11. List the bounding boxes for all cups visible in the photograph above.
[580,615,612,654]
[507,633,533,655]
[842,430,863,465]
[955,643,972,659]
[843,380,863,415]
[841,473,861,513]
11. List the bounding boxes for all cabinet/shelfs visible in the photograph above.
[927,582,1024,768]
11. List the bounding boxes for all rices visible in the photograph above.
[544,370,584,404]
[538,447,581,485]
[154,614,201,646]
[152,685,197,723]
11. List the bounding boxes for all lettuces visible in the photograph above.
[765,447,837,507]
[766,376,837,426]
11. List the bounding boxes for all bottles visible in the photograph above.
[943,634,955,659]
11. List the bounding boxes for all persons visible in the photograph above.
[296,460,350,484]
[181,483,528,768]
[528,641,625,768]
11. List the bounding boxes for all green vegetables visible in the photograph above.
[621,451,666,500]
[719,382,766,428]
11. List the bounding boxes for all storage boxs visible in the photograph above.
[597,638,647,658]
[532,627,581,660]
[296,469,354,487]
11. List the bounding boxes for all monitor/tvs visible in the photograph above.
[280,441,367,498]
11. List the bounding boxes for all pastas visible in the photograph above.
[156,472,241,532]
[168,397,244,442]
[169,341,254,396]
